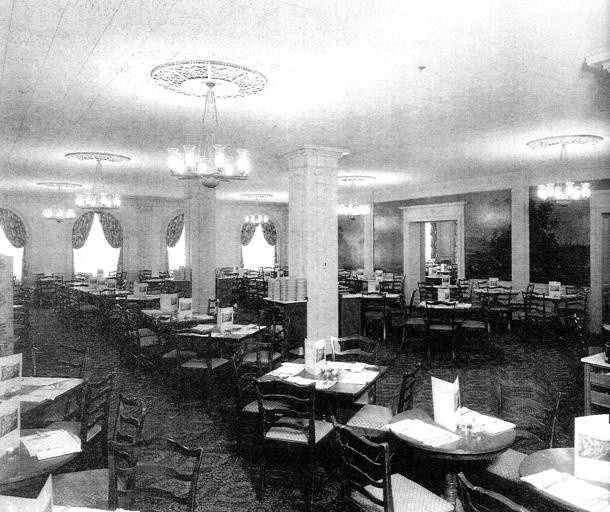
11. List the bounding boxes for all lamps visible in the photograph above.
[527,132,604,206]
[336,177,384,221]
[66,151,132,214]
[36,181,81,225]
[240,193,277,228]
[149,60,266,191]
